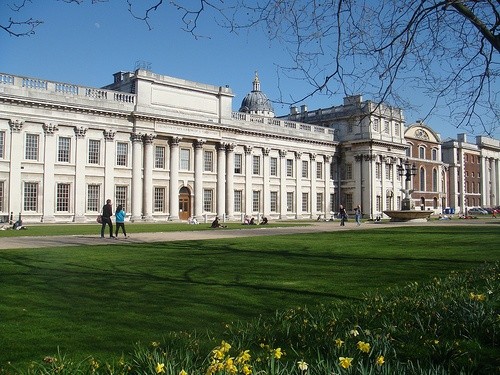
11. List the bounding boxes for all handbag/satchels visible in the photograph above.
[97,215,102,224]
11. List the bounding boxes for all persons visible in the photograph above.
[13,220,28,230]
[339,205,346,226]
[492,208,497,218]
[354,204,361,227]
[101,199,115,239]
[260,217,267,225]
[115,205,129,239]
[210,217,223,228]
[330,216,334,221]
[459,215,465,218]
[317,215,322,221]
[250,218,254,225]
[439,213,448,218]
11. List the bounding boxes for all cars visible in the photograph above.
[468,206,500,216]
